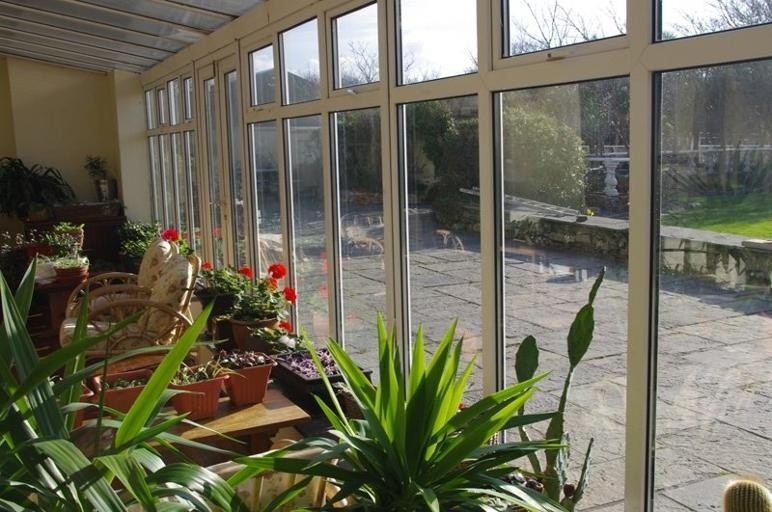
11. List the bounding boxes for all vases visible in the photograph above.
[193,288,240,332]
[229,319,280,350]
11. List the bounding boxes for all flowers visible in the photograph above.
[180,260,297,320]
[118,220,191,259]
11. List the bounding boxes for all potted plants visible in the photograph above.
[72,351,278,430]
[268,347,373,413]
[0,155,121,286]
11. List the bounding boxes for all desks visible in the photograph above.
[80,390,312,489]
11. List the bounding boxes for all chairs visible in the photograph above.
[60,236,202,366]
